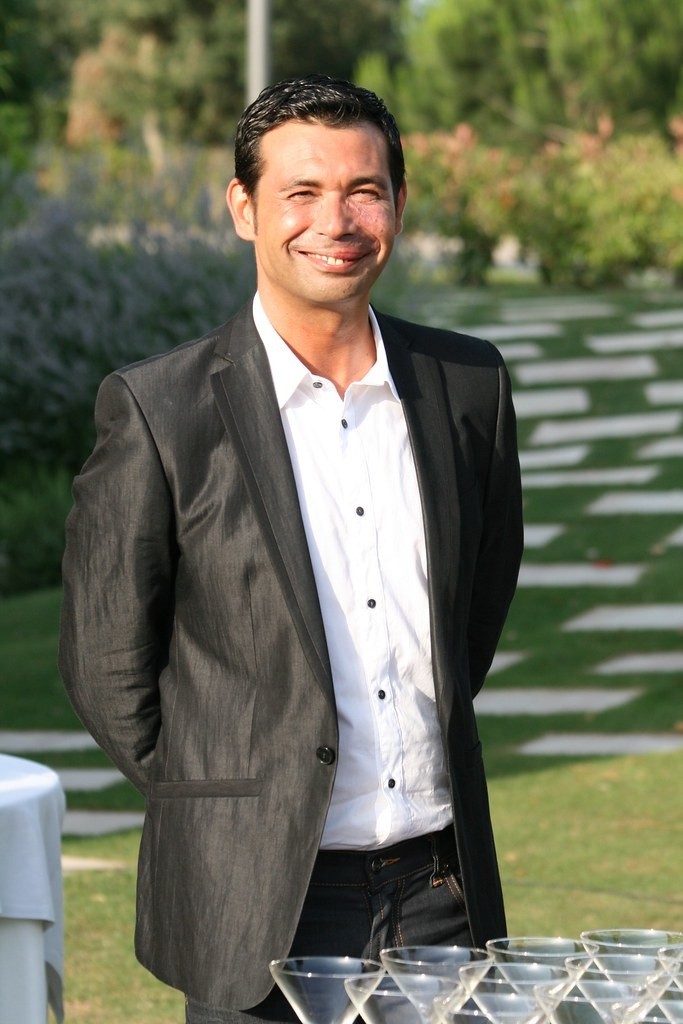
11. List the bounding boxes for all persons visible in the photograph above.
[60,76,524,1024]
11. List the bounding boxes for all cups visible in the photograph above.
[268,929,683,1024]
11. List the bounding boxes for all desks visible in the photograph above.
[0,750,67,1024]
